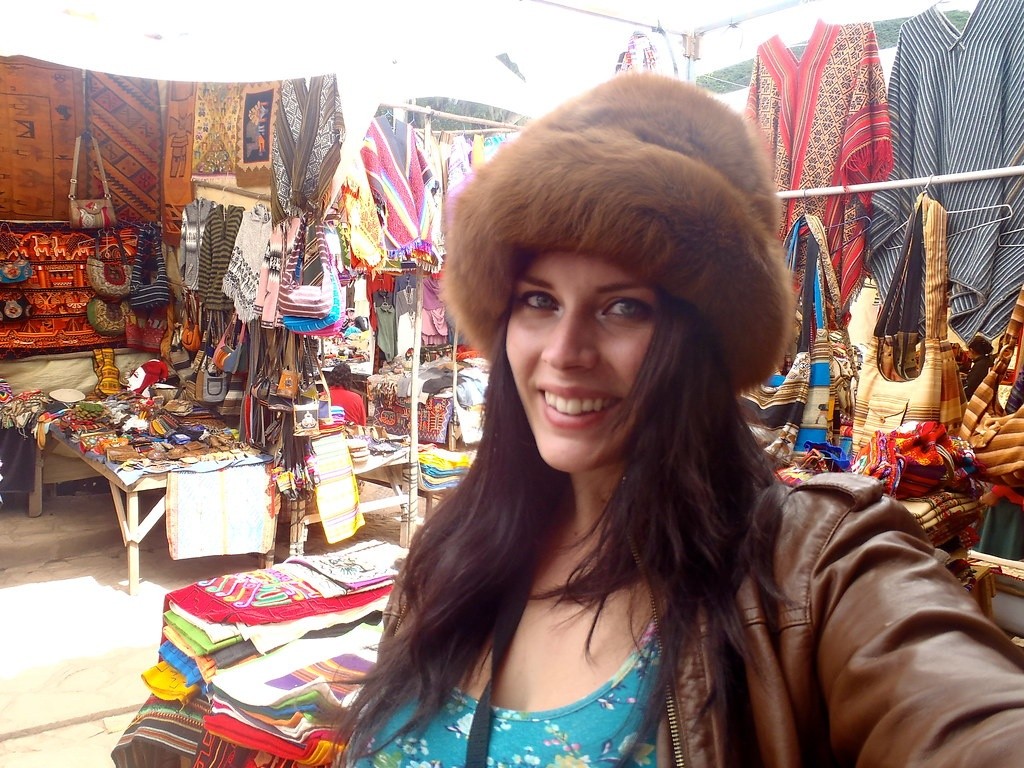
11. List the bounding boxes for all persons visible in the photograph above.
[318,365,366,425]
[964,335,999,402]
[332,72,1024,768]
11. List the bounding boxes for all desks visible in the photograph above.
[26,407,281,595]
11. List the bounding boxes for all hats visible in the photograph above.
[442,74,796,397]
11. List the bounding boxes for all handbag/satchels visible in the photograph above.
[0,283,33,322]
[130,223,170,310]
[125,308,169,352]
[735,194,1024,486]
[87,297,131,335]
[0,259,32,283]
[86,234,132,300]
[161,211,345,437]
[0,223,19,262]
[68,199,116,229]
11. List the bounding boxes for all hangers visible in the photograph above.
[379,102,488,139]
[792,188,873,269]
[881,175,1013,249]
